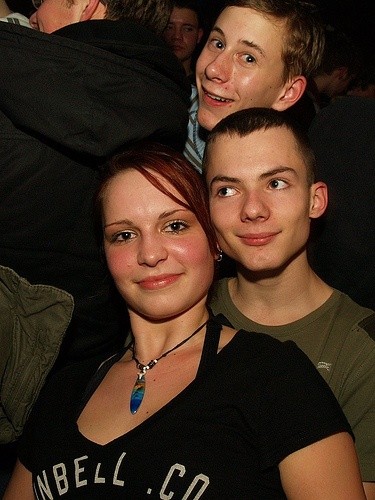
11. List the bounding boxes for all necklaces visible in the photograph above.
[125,322,210,415]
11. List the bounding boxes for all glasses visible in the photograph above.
[32,0,42,9]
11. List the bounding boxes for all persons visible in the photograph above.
[310,64,374,309]
[198,107,374,500]
[194,2,327,135]
[159,3,205,65]
[0,144,367,500]
[0,0,191,468]
